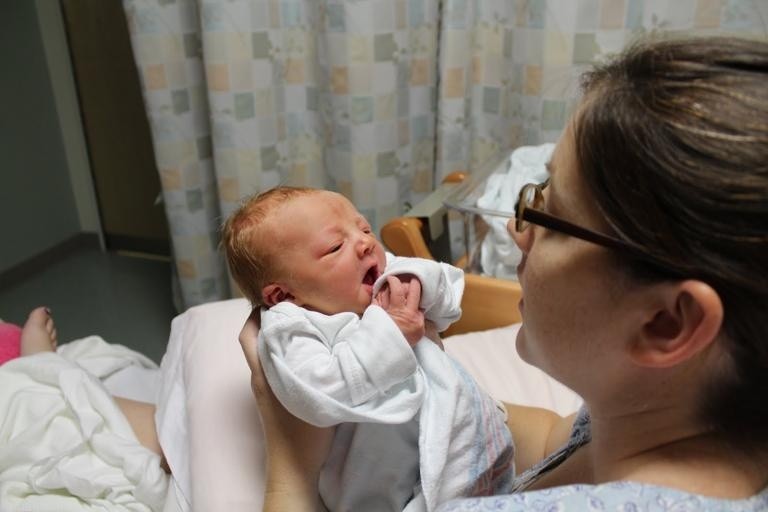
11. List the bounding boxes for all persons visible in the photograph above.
[214,185,515,511]
[19,38,767,512]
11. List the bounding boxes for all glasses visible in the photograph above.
[514,176,683,280]
